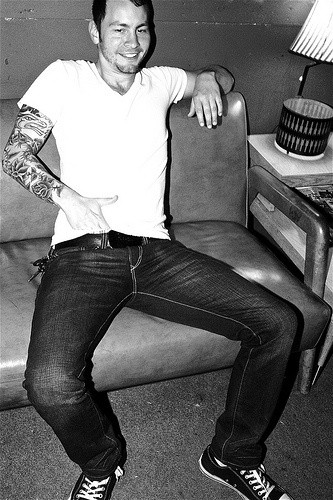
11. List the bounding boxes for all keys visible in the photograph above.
[28,254,48,283]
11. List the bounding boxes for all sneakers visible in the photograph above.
[198,443,293,500]
[68,465,123,500]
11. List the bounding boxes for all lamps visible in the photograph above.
[274,0,333,98]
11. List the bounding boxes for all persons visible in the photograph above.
[2,0,299,500]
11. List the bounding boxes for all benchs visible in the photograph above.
[0,92,332,411]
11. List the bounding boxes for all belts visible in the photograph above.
[48,231,161,257]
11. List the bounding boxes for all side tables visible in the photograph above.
[247,132,333,385]
